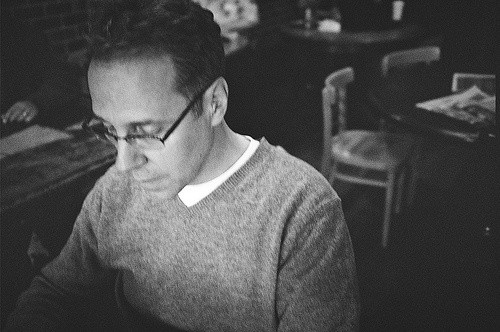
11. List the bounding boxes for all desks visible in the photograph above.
[361,67,500,208]
[276,19,435,52]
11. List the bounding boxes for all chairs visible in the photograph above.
[321,46,496,247]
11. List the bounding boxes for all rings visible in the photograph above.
[23,112,27,118]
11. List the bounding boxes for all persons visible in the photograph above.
[0,0,362,331]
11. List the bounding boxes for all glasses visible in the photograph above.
[82,77,216,149]
